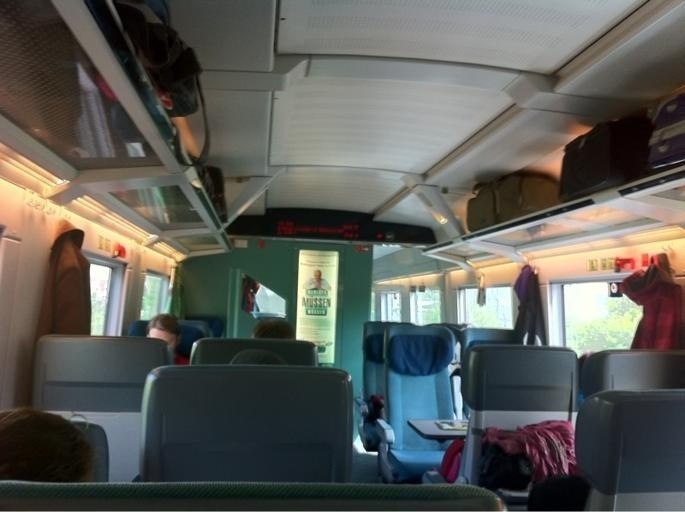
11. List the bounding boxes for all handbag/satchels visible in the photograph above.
[647,93,685,184]
[467,171,559,232]
[143,23,199,118]
[559,115,651,210]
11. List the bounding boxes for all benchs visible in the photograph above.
[0,482,507,512]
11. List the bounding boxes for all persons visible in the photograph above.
[145,314,190,366]
[250,317,293,340]
[304,270,331,291]
[0,405,98,483]
